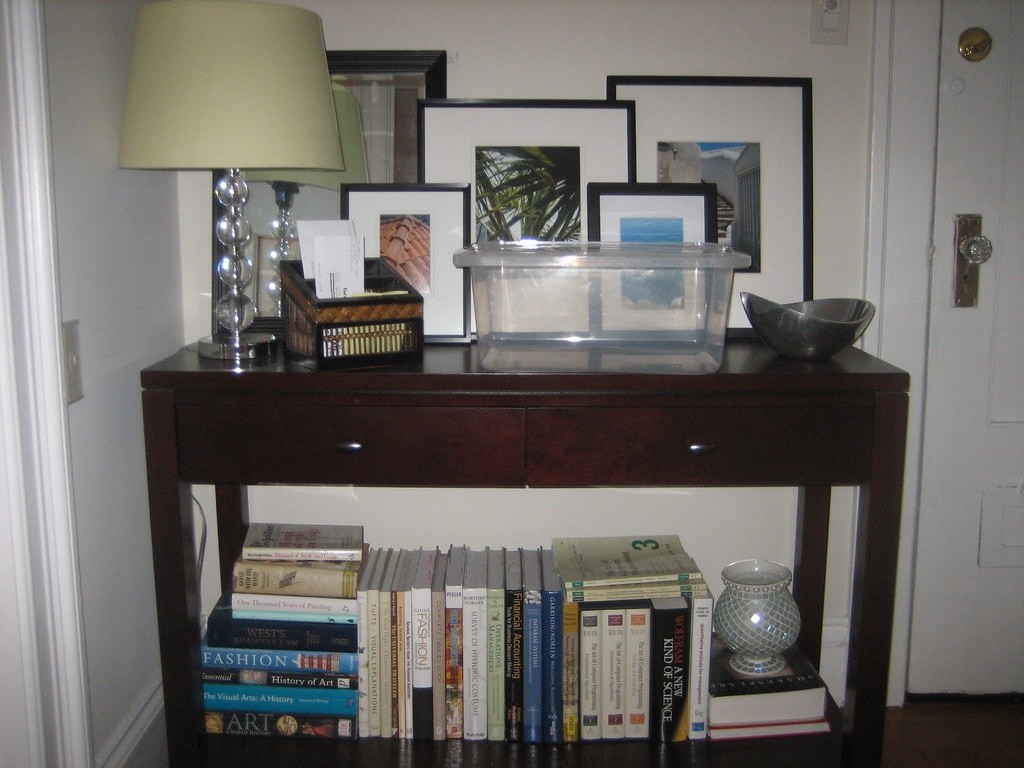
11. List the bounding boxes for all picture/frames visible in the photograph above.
[606,75,814,340]
[340,182,472,342]
[212,49,448,340]
[417,100,636,342]
[587,182,718,346]
[251,234,301,318]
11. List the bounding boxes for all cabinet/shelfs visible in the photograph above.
[140,343,910,768]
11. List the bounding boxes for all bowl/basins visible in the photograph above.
[738,290,875,361]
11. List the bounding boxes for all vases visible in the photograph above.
[714,558,801,676]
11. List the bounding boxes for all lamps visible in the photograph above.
[118,1,344,361]
[240,82,370,318]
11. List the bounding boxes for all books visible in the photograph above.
[202,520,833,743]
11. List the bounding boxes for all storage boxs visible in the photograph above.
[454,241,752,374]
